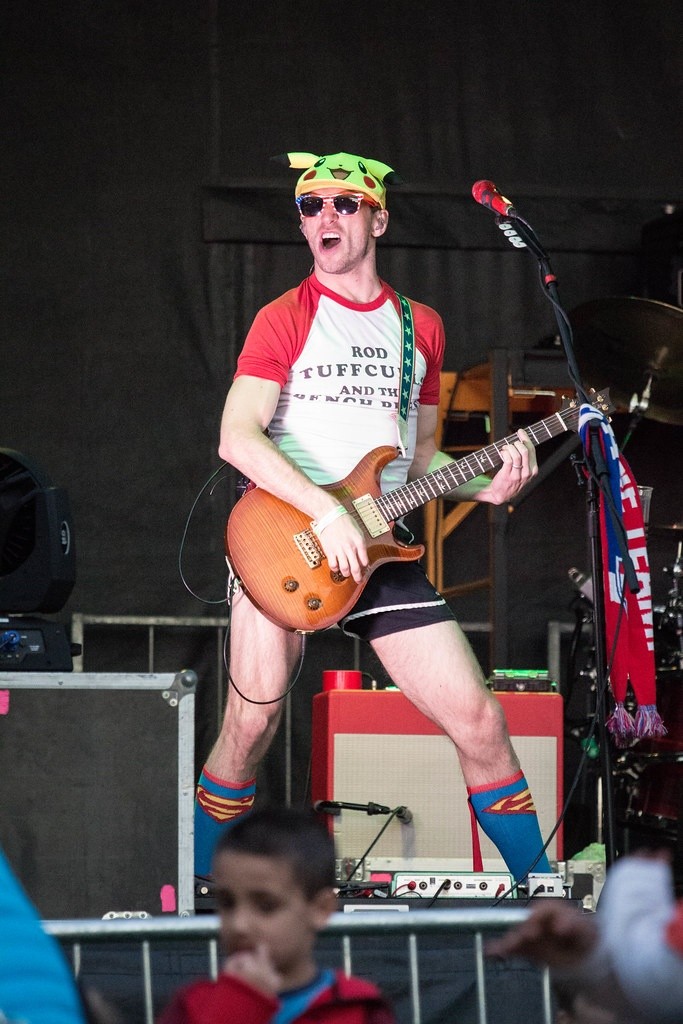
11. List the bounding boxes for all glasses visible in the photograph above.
[295,191,381,218]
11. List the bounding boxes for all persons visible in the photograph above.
[157,807,402,1024]
[488,830,681,1023]
[190,154,566,899]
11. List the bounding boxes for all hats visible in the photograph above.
[275,151,402,209]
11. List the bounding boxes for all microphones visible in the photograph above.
[470,179,519,219]
[396,806,412,824]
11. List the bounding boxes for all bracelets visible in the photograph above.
[311,504,348,535]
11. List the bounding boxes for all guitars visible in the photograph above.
[225,387,618,637]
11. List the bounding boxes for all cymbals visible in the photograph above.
[648,521,683,539]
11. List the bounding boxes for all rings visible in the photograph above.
[511,464,525,470]
